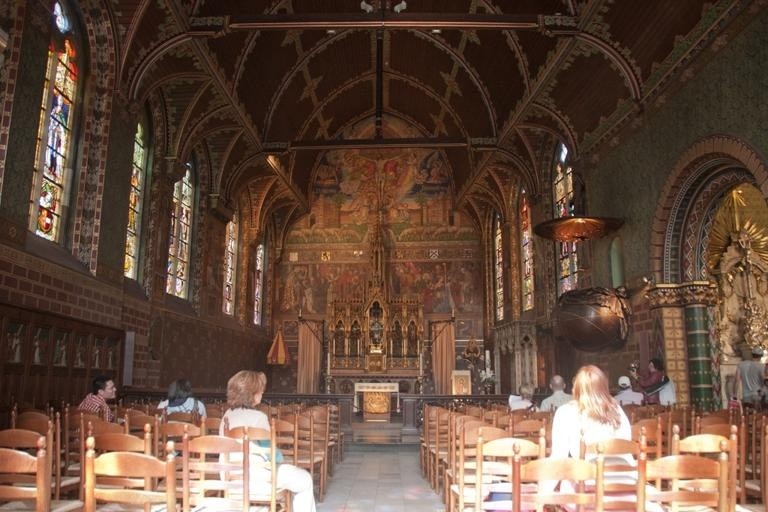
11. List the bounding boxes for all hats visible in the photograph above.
[618,376,631,388]
[752,346,763,355]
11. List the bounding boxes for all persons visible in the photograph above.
[628,357,664,407]
[613,375,644,407]
[293,264,342,312]
[392,263,482,316]
[354,152,405,206]
[155,376,207,461]
[218,369,316,512]
[543,363,638,509]
[72,374,126,462]
[735,347,768,416]
[540,374,573,413]
[49,95,66,178]
[509,383,539,437]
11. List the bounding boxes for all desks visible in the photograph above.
[351,381,401,423]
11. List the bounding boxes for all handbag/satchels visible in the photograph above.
[645,376,669,396]
